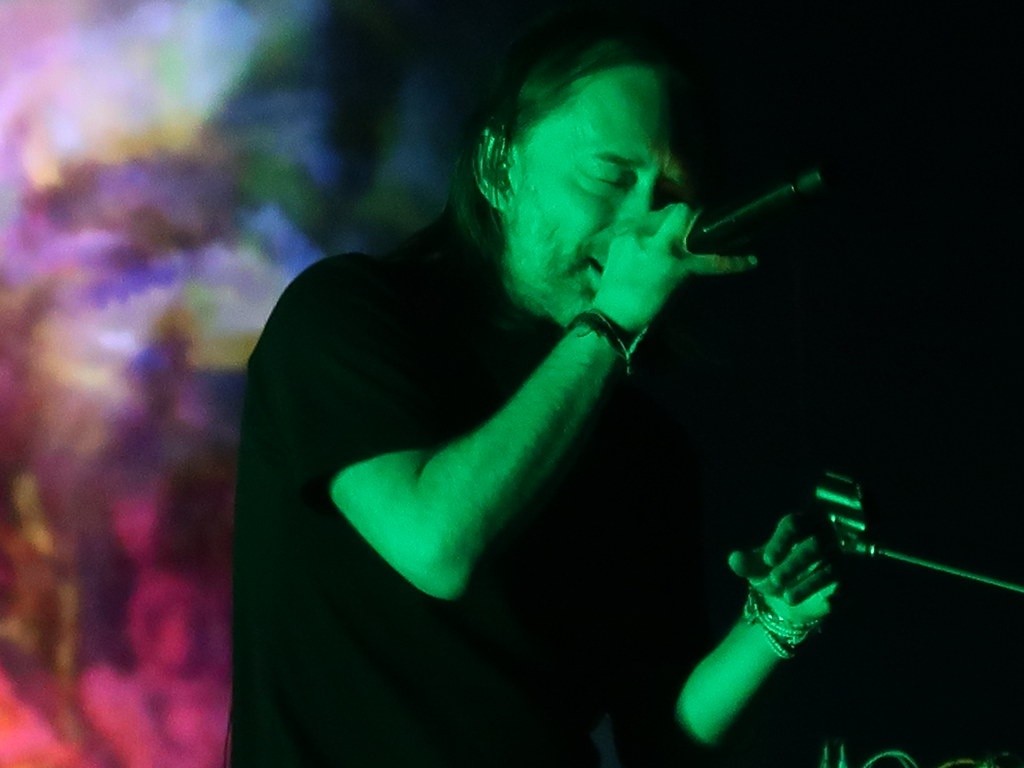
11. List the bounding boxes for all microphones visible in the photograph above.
[687,168,831,257]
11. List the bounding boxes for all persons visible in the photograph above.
[222,0,847,768]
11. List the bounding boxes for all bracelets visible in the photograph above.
[565,306,645,383]
[743,590,819,660]
